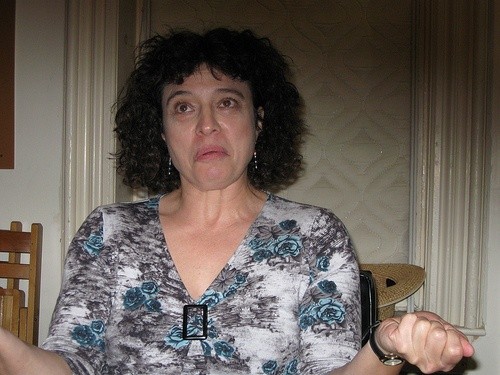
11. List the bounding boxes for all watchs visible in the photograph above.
[370,326,403,366]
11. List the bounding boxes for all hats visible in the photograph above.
[357,263,426,322]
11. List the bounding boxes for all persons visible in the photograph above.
[0,22,475,375]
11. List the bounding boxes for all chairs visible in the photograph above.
[0,223,42,347]
